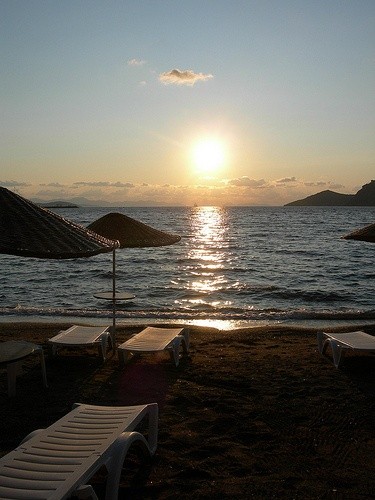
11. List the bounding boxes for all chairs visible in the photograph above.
[0,340,48,396]
[48,324,114,365]
[317,331,375,367]
[0,403,160,500]
[122,327,189,370]
